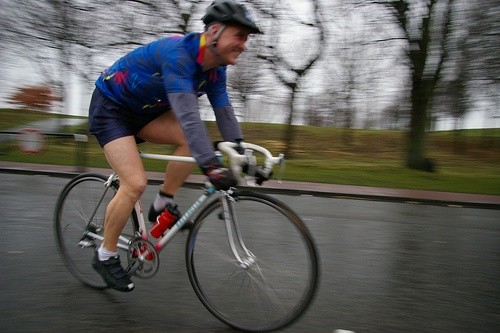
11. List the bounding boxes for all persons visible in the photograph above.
[88,0,273,291]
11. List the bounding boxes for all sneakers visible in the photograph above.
[92,249,135,292]
[147,204,192,231]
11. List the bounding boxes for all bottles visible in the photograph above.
[149,204,179,239]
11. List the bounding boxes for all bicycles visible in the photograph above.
[53,138,321,333]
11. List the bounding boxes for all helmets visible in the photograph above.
[202,0,264,34]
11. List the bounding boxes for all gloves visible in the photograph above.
[205,160,236,191]
